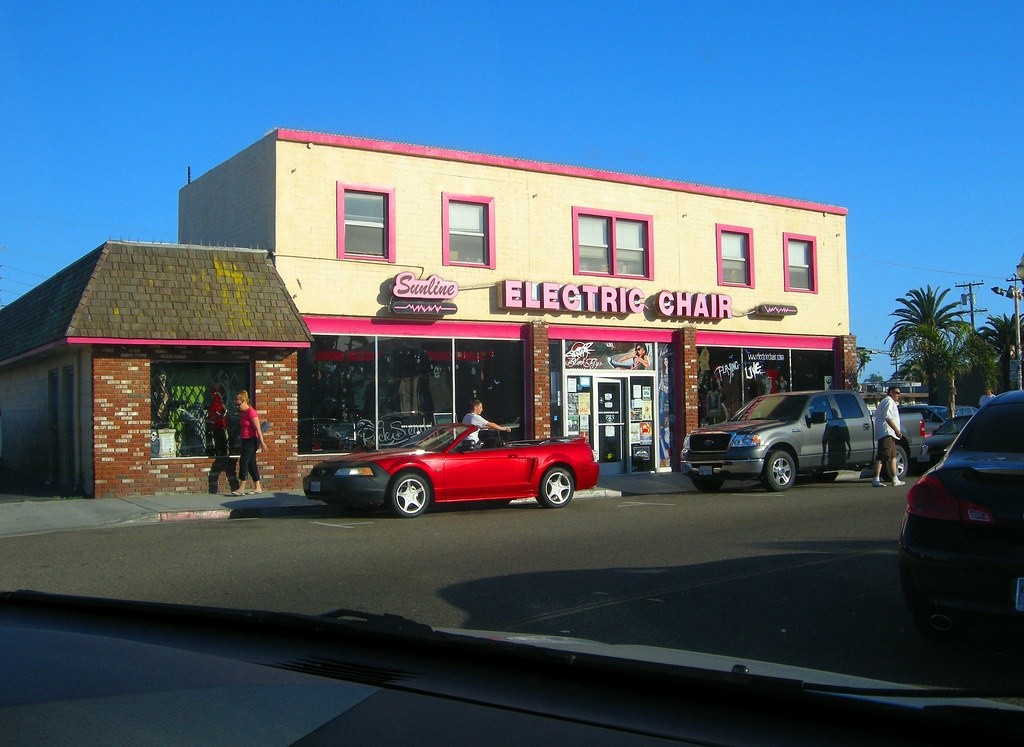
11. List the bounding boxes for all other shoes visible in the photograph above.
[231,491,245,496]
[248,490,262,494]
[872,482,886,487]
[893,481,905,486]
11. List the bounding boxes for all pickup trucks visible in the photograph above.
[681,387,933,493]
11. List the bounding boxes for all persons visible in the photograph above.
[462,400,512,450]
[978,386,996,407]
[231,390,268,496]
[617,343,649,371]
[872,385,905,486]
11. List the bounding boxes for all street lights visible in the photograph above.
[991,285,1023,390]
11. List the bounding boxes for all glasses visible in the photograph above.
[636,347,642,351]
[894,391,900,394]
[236,402,243,406]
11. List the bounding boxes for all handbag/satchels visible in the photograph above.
[237,436,261,451]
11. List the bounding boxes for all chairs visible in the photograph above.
[481,437,502,448]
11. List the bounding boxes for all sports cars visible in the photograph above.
[303,419,599,518]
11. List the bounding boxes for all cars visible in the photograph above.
[895,404,980,470]
[376,411,448,443]
[298,417,364,455]
[898,385,1024,641]
